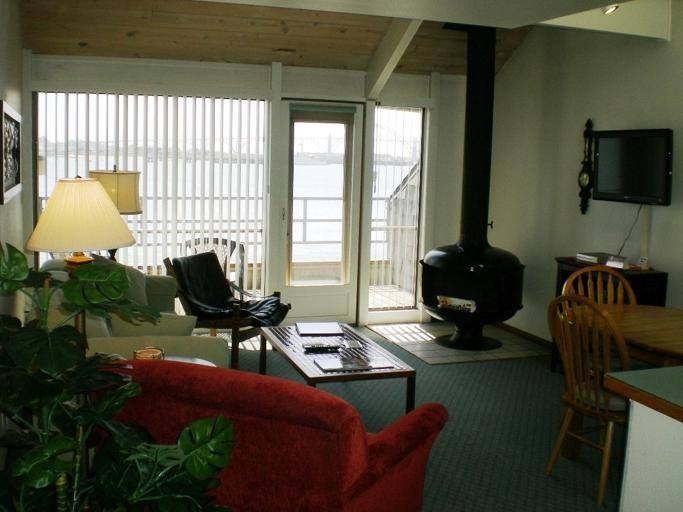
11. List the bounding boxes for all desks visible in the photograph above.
[562,304,683,460]
[556,257,668,352]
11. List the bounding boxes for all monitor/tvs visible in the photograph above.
[592,128,675,205]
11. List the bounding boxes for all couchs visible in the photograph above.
[90,360,448,512]
[13,259,229,368]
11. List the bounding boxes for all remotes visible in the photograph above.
[302,342,341,351]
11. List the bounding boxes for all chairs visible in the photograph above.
[163,250,291,374]
[542,295,631,510]
[561,265,637,310]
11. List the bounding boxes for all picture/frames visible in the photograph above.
[0,100,22,205]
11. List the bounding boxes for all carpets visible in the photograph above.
[366,323,552,365]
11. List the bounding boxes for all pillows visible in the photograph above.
[85,254,197,337]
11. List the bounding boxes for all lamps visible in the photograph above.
[88,165,142,262]
[601,4,619,15]
[26,176,135,464]
